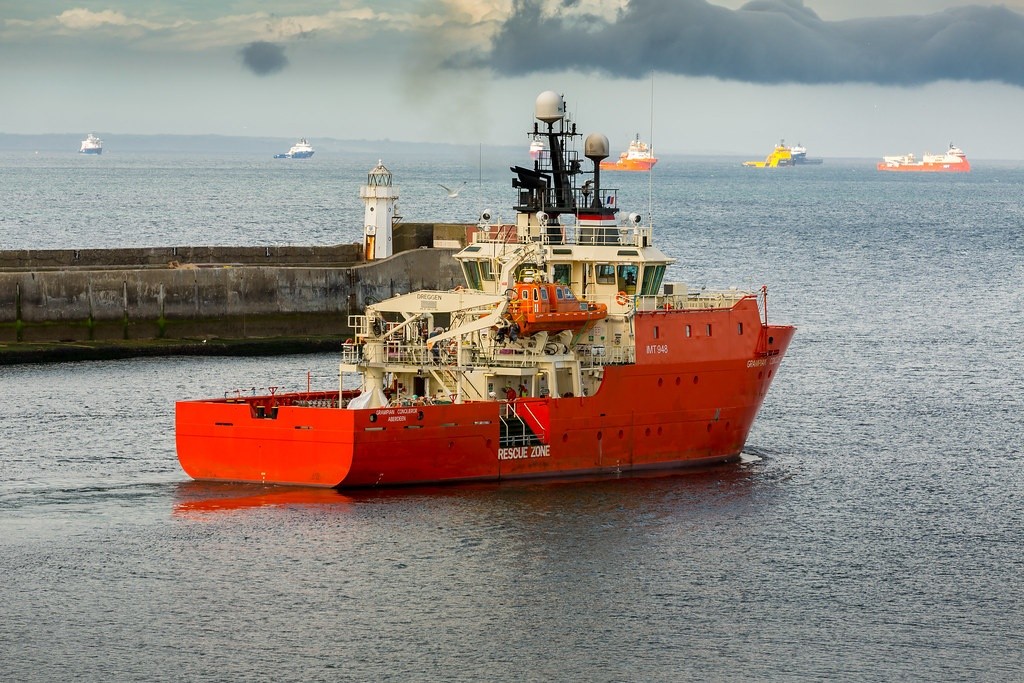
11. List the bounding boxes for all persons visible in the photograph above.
[430,327,444,365]
[519,383,529,397]
[502,384,518,414]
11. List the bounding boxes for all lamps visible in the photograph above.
[629,212,641,227]
[482,209,493,223]
[536,211,550,226]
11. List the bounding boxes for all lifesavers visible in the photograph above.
[455,286,465,292]
[615,290,628,305]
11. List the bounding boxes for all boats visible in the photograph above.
[273,138,314,159]
[875,143,969,173]
[743,140,822,167]
[599,133,657,171]
[79,135,102,153]
[176,74,797,491]
[530,139,545,160]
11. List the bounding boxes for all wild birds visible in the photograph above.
[438,182,466,198]
